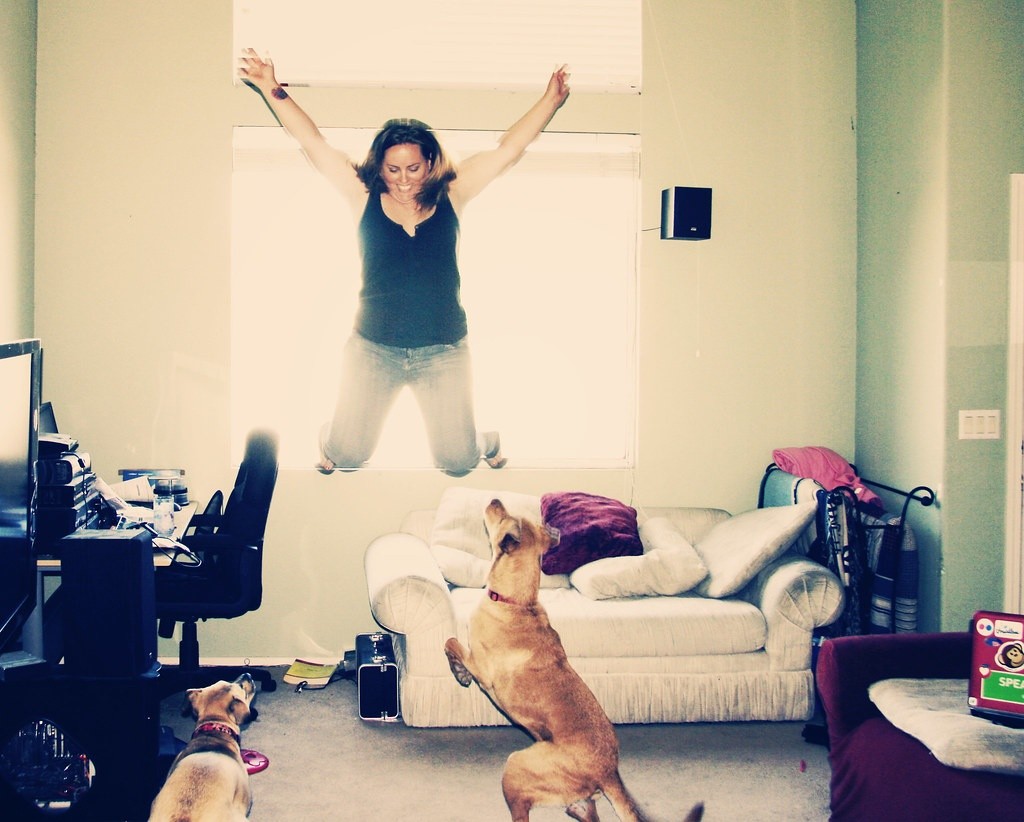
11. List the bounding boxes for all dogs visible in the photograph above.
[147,672,257,822]
[444,499,706,821]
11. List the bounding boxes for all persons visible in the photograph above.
[237,45,572,479]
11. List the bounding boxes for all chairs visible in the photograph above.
[152,429,280,694]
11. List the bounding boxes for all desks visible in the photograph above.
[38,499,198,577]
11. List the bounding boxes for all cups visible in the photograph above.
[154,496,174,536]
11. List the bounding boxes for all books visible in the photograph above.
[284,659,335,686]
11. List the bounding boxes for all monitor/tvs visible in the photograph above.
[0,336,39,649]
[40,402,59,435]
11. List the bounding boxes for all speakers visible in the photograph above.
[660,186,712,241]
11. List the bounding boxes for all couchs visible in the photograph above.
[367,509,841,729]
[817,633,1024,822]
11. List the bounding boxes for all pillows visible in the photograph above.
[541,492,641,577]
[433,483,570,590]
[695,501,817,599]
[572,509,708,600]
